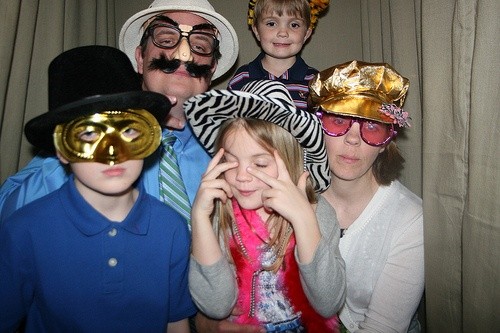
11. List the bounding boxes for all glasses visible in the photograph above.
[316,111,394,147]
[140,24,220,56]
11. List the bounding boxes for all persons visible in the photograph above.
[227,0,330,114]
[194,60,424,333]
[182,80,346,333]
[0,0,240,237]
[0,45,195,333]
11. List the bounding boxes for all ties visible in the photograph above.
[159,133,191,233]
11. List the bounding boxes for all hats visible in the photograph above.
[308,60,411,124]
[183,80,331,194]
[119,0,239,80]
[24,45,172,156]
[247,0,330,26]
